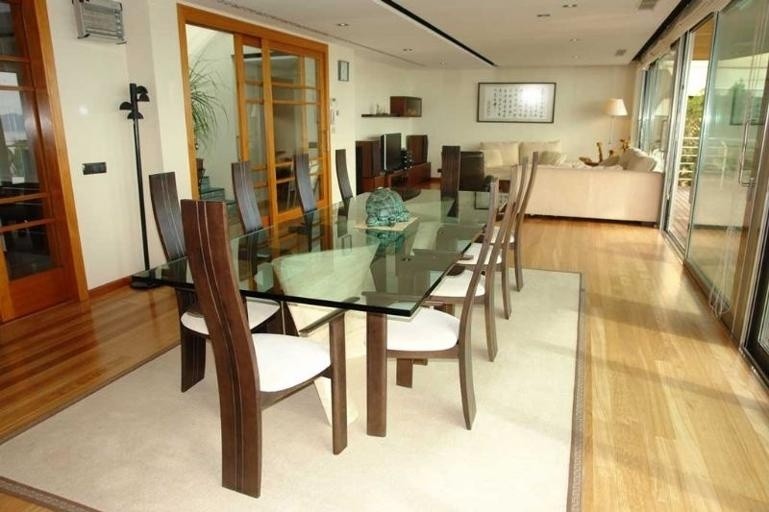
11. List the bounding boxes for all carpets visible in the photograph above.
[2,264,588,512]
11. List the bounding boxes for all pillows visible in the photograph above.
[534,150,624,172]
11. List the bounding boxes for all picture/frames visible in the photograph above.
[339,60,349,82]
[477,82,555,123]
[727,76,769,126]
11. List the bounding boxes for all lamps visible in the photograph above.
[653,97,674,153]
[119,82,172,291]
[603,98,628,146]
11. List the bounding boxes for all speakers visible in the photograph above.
[356,140,381,177]
[406,134,427,163]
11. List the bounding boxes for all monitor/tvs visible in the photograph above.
[380,132,401,171]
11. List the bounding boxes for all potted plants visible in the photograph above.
[183,46,231,190]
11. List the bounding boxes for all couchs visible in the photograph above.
[473,141,665,226]
[691,169,752,231]
